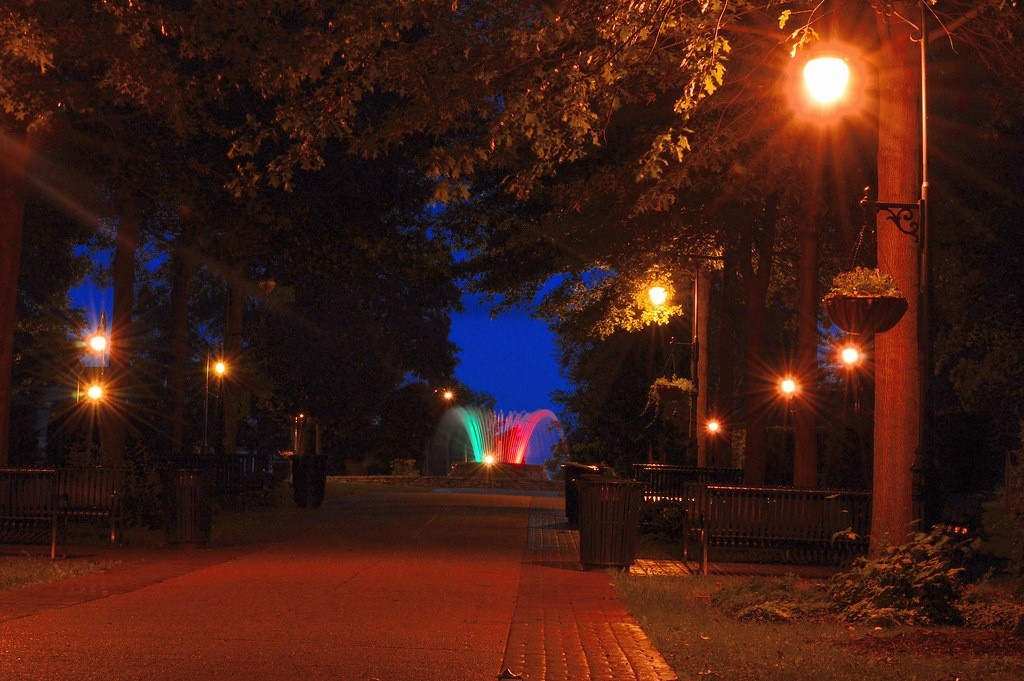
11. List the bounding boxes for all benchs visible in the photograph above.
[632,465,870,575]
[0,453,267,560]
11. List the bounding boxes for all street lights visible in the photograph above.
[648,259,699,465]
[203,361,226,453]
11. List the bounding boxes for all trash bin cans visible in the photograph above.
[159,464,213,547]
[571,477,643,565]
[289,453,329,507]
[560,462,599,526]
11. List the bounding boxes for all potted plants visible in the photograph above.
[821,268,910,333]
[641,377,698,429]
